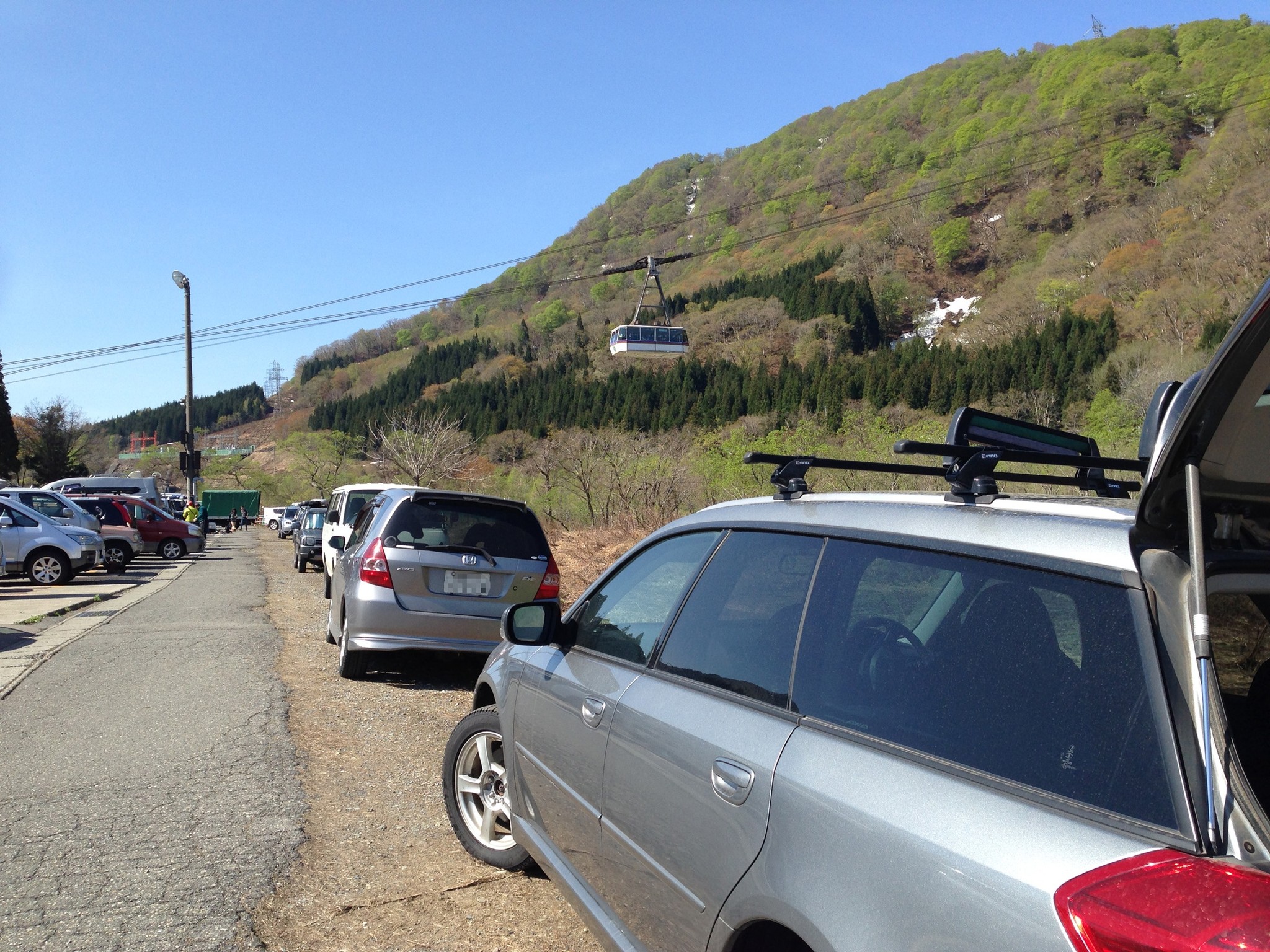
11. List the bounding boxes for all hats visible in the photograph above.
[186,501,193,505]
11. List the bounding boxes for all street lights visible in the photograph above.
[171,271,201,504]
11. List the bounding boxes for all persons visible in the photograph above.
[183,501,199,524]
[228,508,237,532]
[239,506,248,531]
[194,503,207,541]
[611,331,685,344]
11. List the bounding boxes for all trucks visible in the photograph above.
[201,490,261,526]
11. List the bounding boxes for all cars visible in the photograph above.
[323,488,564,675]
[0,492,205,585]
[263,498,325,573]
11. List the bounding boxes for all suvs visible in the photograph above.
[441,270,1270,952]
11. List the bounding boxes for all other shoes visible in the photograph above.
[239,529,242,530]
[245,529,247,530]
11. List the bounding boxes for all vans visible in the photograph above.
[39,473,165,511]
[322,482,422,596]
